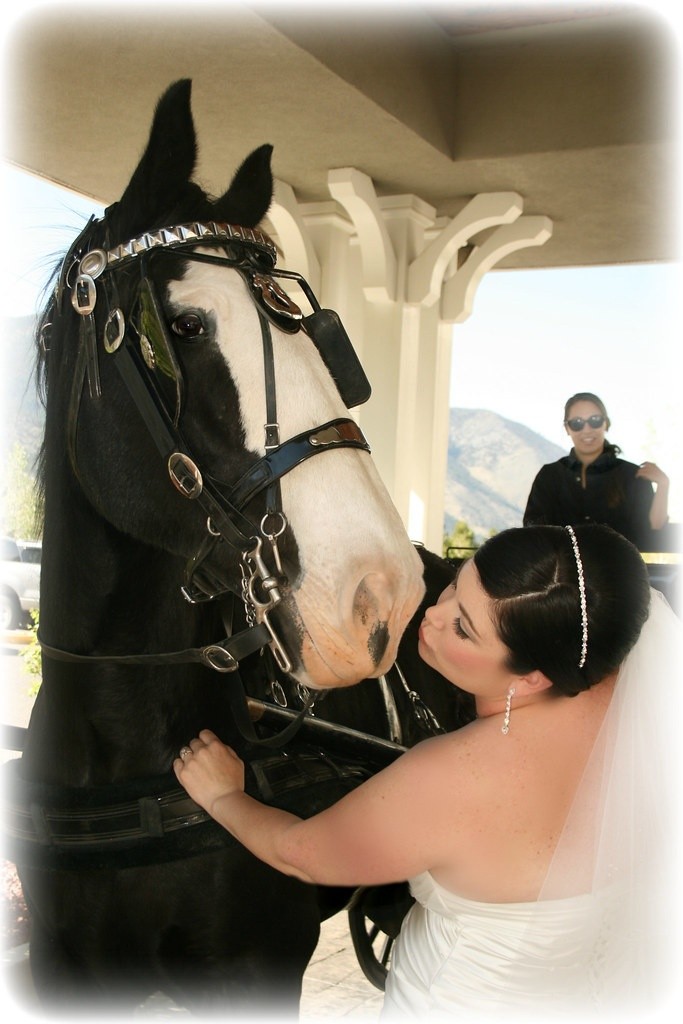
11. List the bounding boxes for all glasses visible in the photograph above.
[564,414,606,432]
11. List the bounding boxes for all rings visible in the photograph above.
[181,748,192,760]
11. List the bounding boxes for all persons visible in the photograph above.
[173,518,680,1019]
[522,392,670,552]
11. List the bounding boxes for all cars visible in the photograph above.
[2,537,43,630]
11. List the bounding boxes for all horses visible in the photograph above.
[7,76,475,1019]
